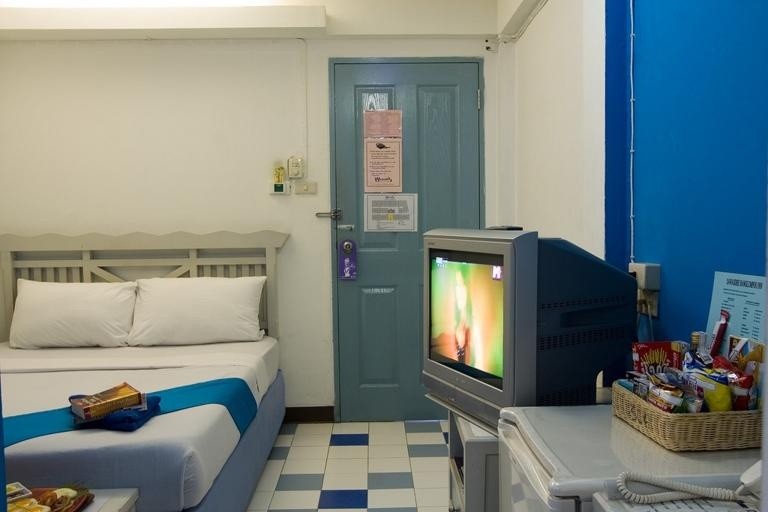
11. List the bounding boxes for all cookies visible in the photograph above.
[37,487,78,512]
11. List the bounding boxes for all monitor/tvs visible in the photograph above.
[420,226,638,438]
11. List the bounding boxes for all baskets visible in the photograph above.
[611,377,759,451]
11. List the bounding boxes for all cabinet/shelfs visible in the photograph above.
[448,409,499,511]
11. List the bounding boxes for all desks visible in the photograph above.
[81,488,140,512]
[591,492,761,512]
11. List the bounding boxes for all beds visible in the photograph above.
[0,231,291,511]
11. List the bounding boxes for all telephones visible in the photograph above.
[740,459,763,504]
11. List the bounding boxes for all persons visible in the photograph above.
[448,262,474,366]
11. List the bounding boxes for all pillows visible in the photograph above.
[10,278,136,348]
[127,276,268,345]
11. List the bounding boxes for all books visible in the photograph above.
[69,381,142,420]
